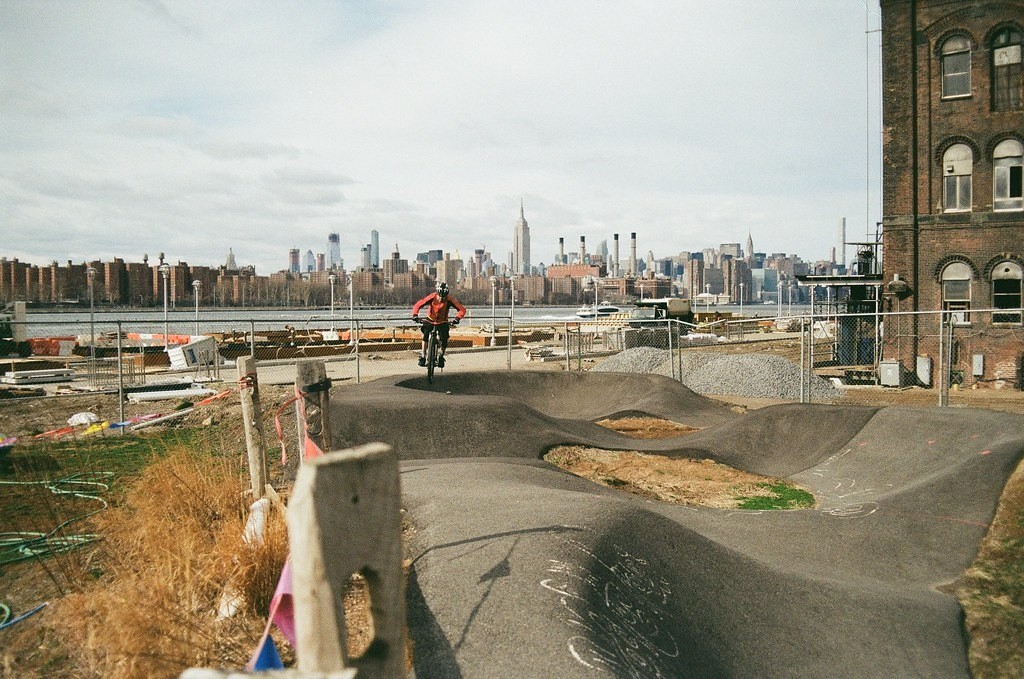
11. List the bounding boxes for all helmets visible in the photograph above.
[437,282,450,297]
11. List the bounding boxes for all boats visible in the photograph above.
[577,300,620,320]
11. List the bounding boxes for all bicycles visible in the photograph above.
[411,314,453,386]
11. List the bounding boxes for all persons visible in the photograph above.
[412,281,466,368]
[285,325,297,339]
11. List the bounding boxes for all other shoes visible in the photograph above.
[438,355,446,368]
[417,355,426,366]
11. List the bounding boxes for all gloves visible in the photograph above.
[453,318,460,325]
[412,316,419,321]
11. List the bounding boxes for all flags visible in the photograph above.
[250,436,326,672]
